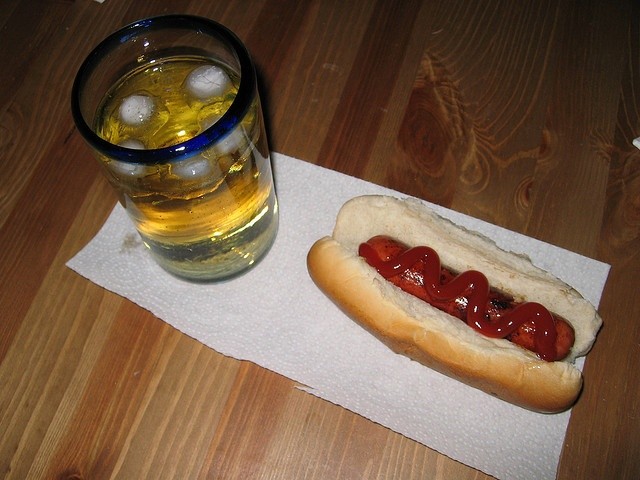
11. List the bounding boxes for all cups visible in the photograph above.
[70,13,280,285]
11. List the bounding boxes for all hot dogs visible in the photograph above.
[303,193,606,416]
